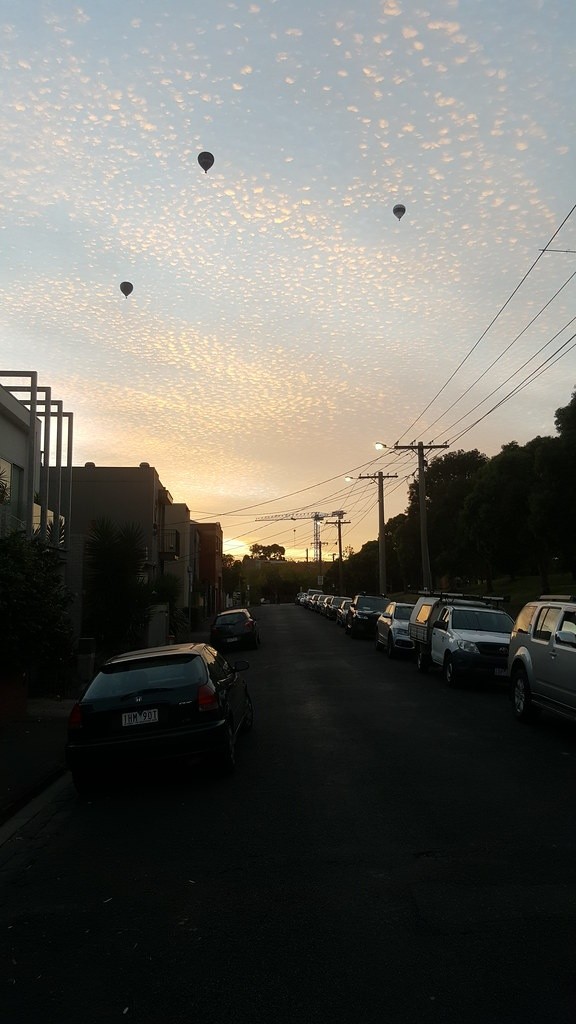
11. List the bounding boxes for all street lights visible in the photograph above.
[373,443,433,592]
[345,475,387,594]
[314,520,344,597]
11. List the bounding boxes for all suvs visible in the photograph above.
[345,591,391,640]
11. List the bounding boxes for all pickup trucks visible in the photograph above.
[406,585,516,688]
[376,602,416,660]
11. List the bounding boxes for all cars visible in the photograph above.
[295,589,353,627]
[64,641,257,800]
[507,592,576,727]
[208,608,259,651]
